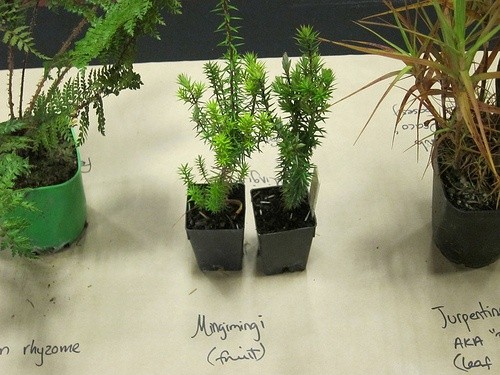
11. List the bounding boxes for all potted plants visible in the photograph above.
[247,23,338,277]
[175,0,278,272]
[311,0,499,270]
[0,1,182,265]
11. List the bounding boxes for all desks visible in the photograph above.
[0,49,497,375]
